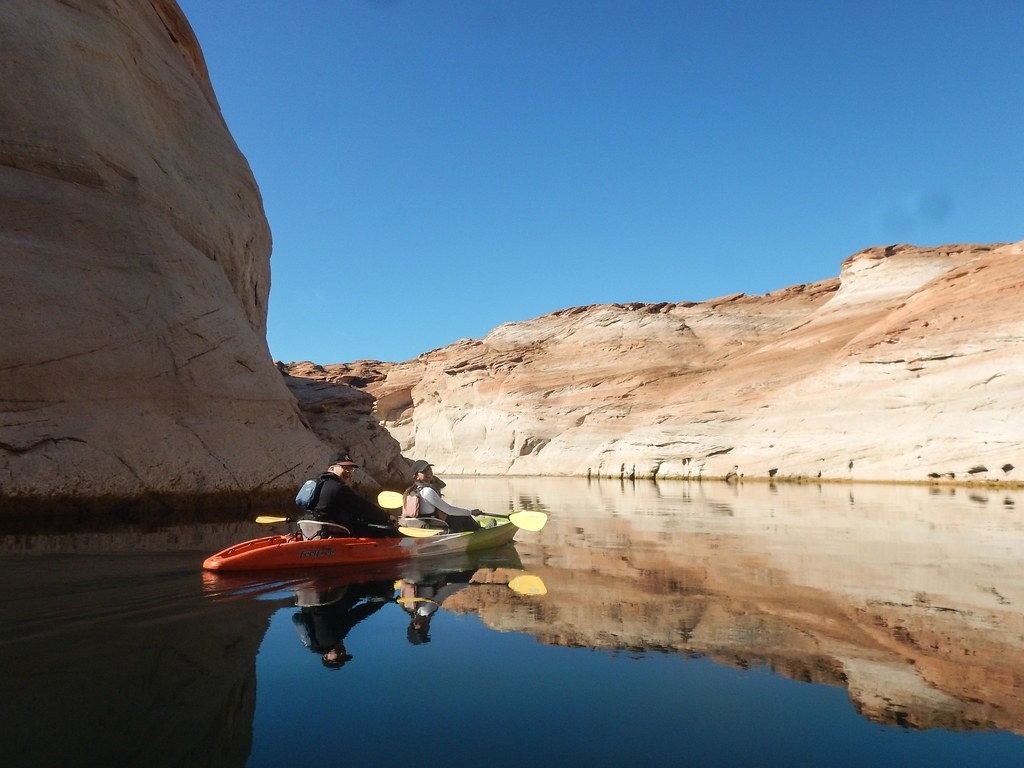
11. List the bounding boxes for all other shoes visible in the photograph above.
[485,518,497,529]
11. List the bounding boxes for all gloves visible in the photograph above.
[472,509,481,516]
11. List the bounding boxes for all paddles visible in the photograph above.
[377,490,548,532]
[255,515,445,538]
[365,574,547,606]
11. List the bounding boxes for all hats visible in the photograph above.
[412,460,435,474]
[329,450,359,468]
[322,656,352,669]
[406,626,430,646]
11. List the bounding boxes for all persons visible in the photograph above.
[393,576,469,645]
[304,453,400,538]
[402,460,497,533]
[290,578,396,668]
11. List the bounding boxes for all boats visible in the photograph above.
[200,512,521,572]
